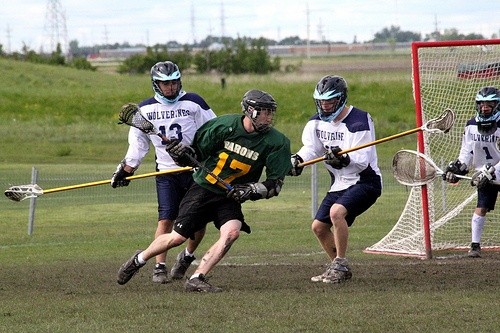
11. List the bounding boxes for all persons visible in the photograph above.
[287,76,382,283]
[118,89,291,291]
[443,87,500,256]
[112,61,217,283]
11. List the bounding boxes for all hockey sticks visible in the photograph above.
[297,107,456,167]
[391,148,473,186]
[3,167,196,202]
[117,102,237,194]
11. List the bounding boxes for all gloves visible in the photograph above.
[323,145,350,170]
[111,159,134,189]
[166,139,196,168]
[286,154,305,178]
[227,182,256,204]
[441,159,468,184]
[470,163,496,188]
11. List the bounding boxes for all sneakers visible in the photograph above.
[184,274,220,293]
[468,242,482,257]
[152,262,169,282]
[171,250,197,278]
[117,250,147,285]
[311,258,352,283]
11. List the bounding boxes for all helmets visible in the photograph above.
[313,75,349,122]
[241,89,279,134]
[475,87,500,125]
[149,60,184,105]
